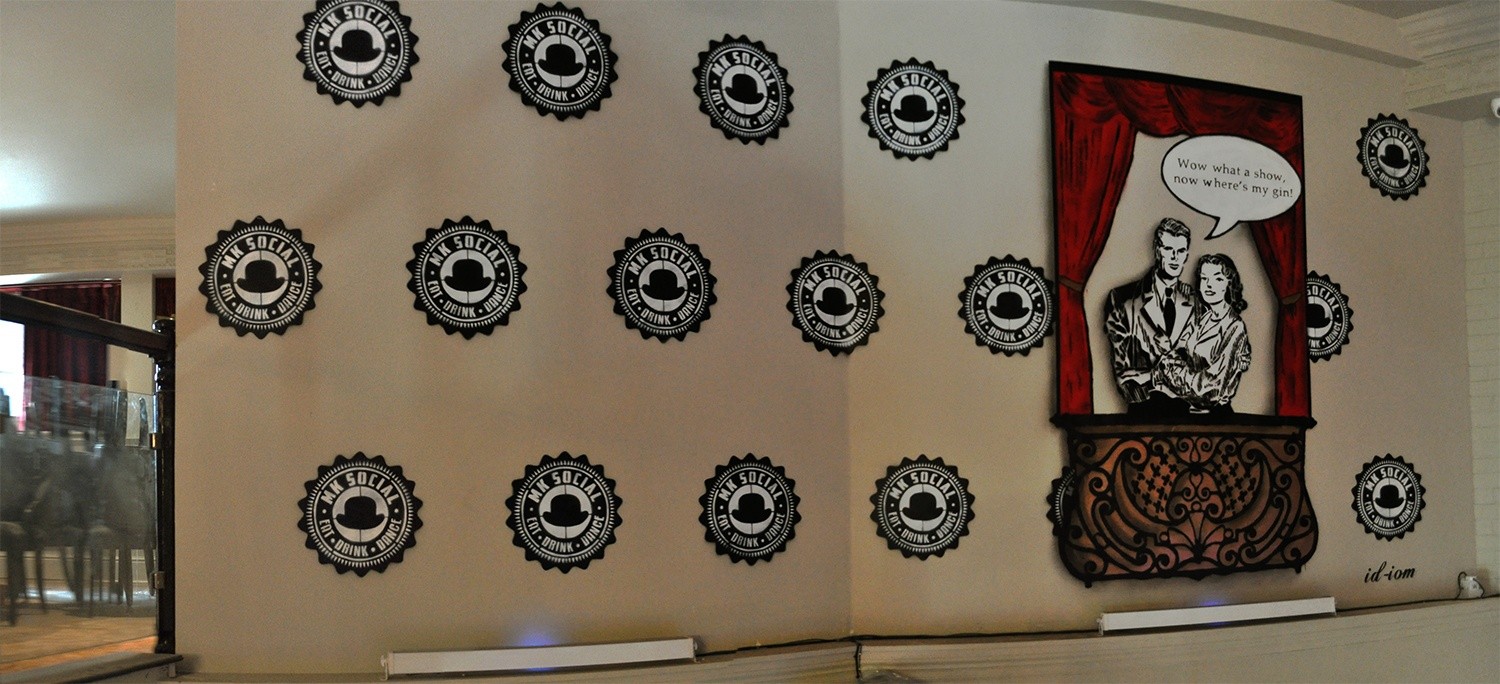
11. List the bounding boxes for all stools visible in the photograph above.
[0,519,157,625]
